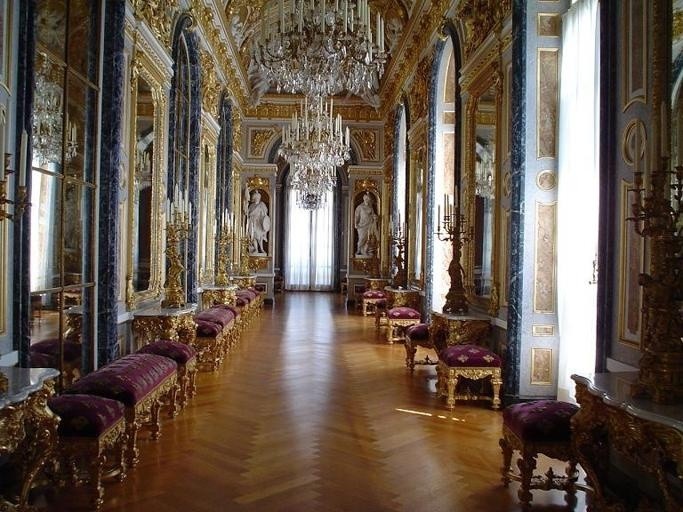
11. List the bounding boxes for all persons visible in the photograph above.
[355,193,378,255]
[246,192,270,253]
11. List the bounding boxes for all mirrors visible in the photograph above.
[128,46,161,299]
[17,21,104,373]
[459,65,505,316]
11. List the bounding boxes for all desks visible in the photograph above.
[133,275,256,345]
[570,371,682,510]
[364,277,492,353]
[0,364,62,510]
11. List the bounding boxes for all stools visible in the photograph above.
[195,286,265,375]
[500,397,589,510]
[27,328,83,390]
[362,290,504,412]
[49,341,196,510]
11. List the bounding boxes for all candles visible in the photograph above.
[162,181,193,226]
[1,113,29,191]
[387,211,410,240]
[435,192,474,235]
[629,99,682,190]
[212,206,255,243]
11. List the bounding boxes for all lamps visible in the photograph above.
[30,67,82,171]
[249,0,390,108]
[279,93,354,212]
